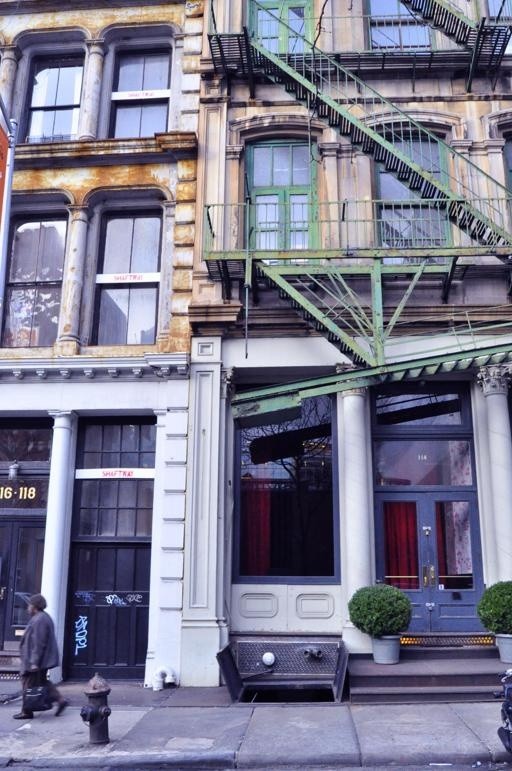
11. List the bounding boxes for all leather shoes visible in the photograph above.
[11,713,35,721]
[53,696,70,718]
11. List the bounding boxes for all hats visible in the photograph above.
[22,594,47,611]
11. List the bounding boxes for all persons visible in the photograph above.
[11,594,72,719]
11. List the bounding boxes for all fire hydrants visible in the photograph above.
[78,670,113,745]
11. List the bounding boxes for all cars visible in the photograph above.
[493,667,512,754]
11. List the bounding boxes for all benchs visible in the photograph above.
[216,636,348,702]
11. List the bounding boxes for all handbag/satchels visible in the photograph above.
[21,684,52,713]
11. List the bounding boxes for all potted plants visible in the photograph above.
[478,579,511,664]
[347,582,412,665]
